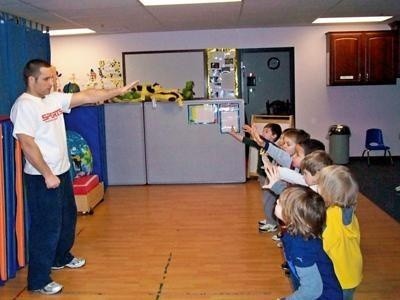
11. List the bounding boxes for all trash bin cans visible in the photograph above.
[329,125,351,164]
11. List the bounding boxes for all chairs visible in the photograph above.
[361,128,394,167]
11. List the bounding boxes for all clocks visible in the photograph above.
[267,57,280,70]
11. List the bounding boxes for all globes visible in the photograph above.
[64,82,80,93]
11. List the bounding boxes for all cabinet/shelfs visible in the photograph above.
[326,32,396,85]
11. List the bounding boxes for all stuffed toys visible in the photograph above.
[111,81,196,101]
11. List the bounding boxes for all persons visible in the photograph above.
[262,164,343,300]
[311,165,364,300]
[227,121,334,279]
[9,58,141,295]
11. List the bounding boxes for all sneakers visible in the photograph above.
[282,262,291,274]
[271,235,281,241]
[277,243,283,247]
[259,220,278,232]
[33,282,63,295]
[51,257,86,270]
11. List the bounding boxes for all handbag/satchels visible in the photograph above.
[328,125,351,135]
